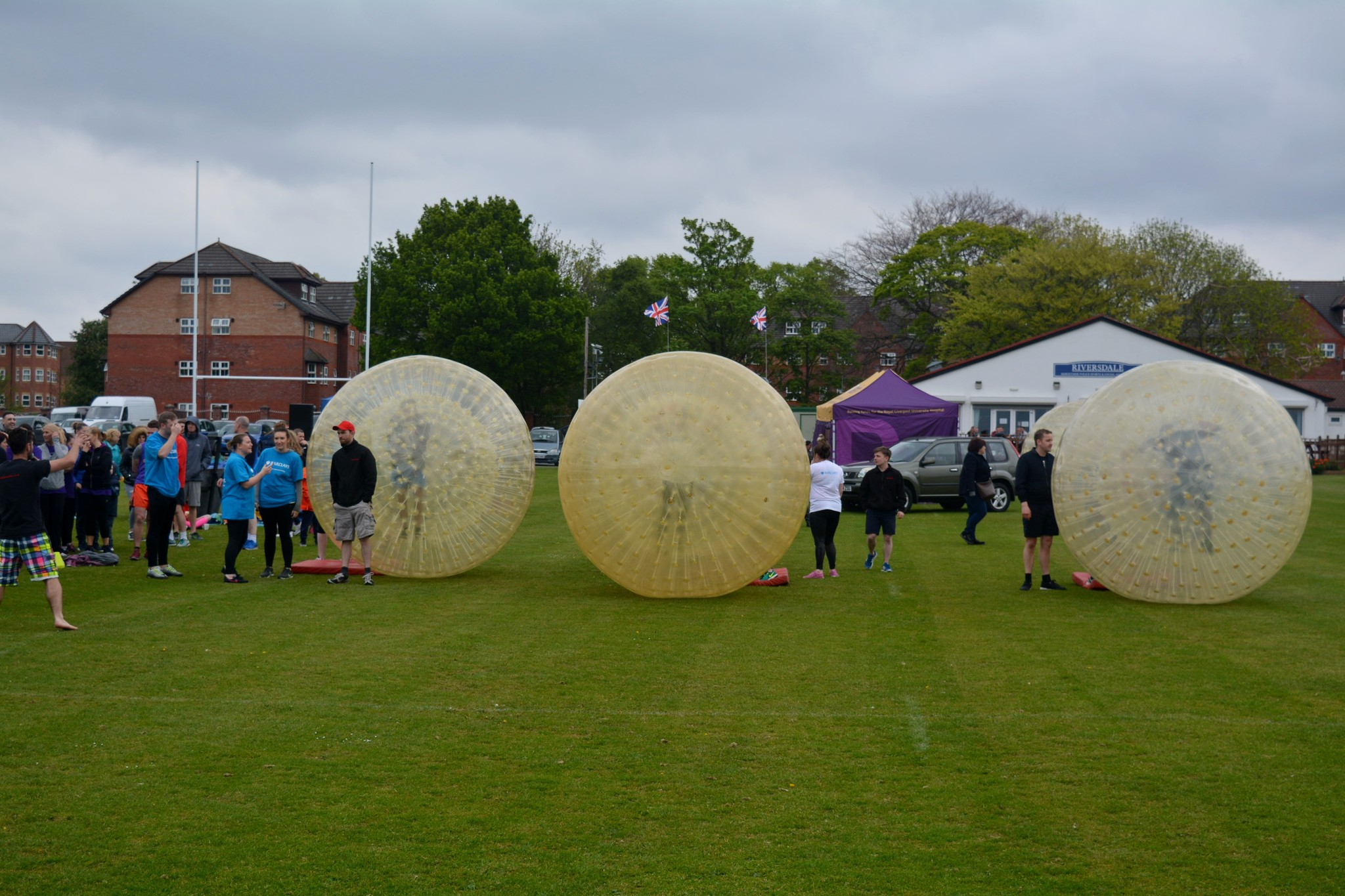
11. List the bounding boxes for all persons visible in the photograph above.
[991,427,1006,438]
[967,426,984,438]
[805,441,813,464]
[958,436,997,545]
[1123,424,1224,556]
[858,445,907,573]
[802,442,845,579]
[634,407,706,551]
[1016,427,1028,445]
[816,433,825,442]
[0,412,378,628]
[387,398,430,541]
[1015,428,1067,592]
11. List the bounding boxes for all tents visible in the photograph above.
[810,367,959,466]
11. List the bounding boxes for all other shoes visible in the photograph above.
[54,540,114,556]
[276,521,318,546]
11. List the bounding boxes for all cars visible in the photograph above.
[0,415,137,453]
[198,419,288,456]
[530,425,570,466]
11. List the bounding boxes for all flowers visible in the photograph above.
[1311,457,1329,469]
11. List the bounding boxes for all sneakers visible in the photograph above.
[865,551,877,569]
[1019,581,1032,591]
[974,539,984,545]
[128,529,204,546]
[162,565,183,577]
[221,567,237,574]
[277,568,293,580]
[327,573,349,584]
[760,571,778,581]
[1040,580,1066,591]
[881,563,892,572]
[829,569,839,578]
[363,572,374,586]
[177,539,191,548]
[224,575,248,583]
[243,540,259,550]
[130,549,140,560]
[960,529,976,544]
[146,566,168,578]
[259,567,274,578]
[803,569,825,579]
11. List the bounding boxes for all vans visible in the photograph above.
[83,396,159,433]
[50,406,90,422]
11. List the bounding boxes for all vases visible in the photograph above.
[1313,468,1324,475]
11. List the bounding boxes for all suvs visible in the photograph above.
[838,434,1021,514]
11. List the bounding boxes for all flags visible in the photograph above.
[749,306,767,332]
[644,296,670,327]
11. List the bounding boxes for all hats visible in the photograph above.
[333,421,355,435]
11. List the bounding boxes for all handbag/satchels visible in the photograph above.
[977,479,995,499]
